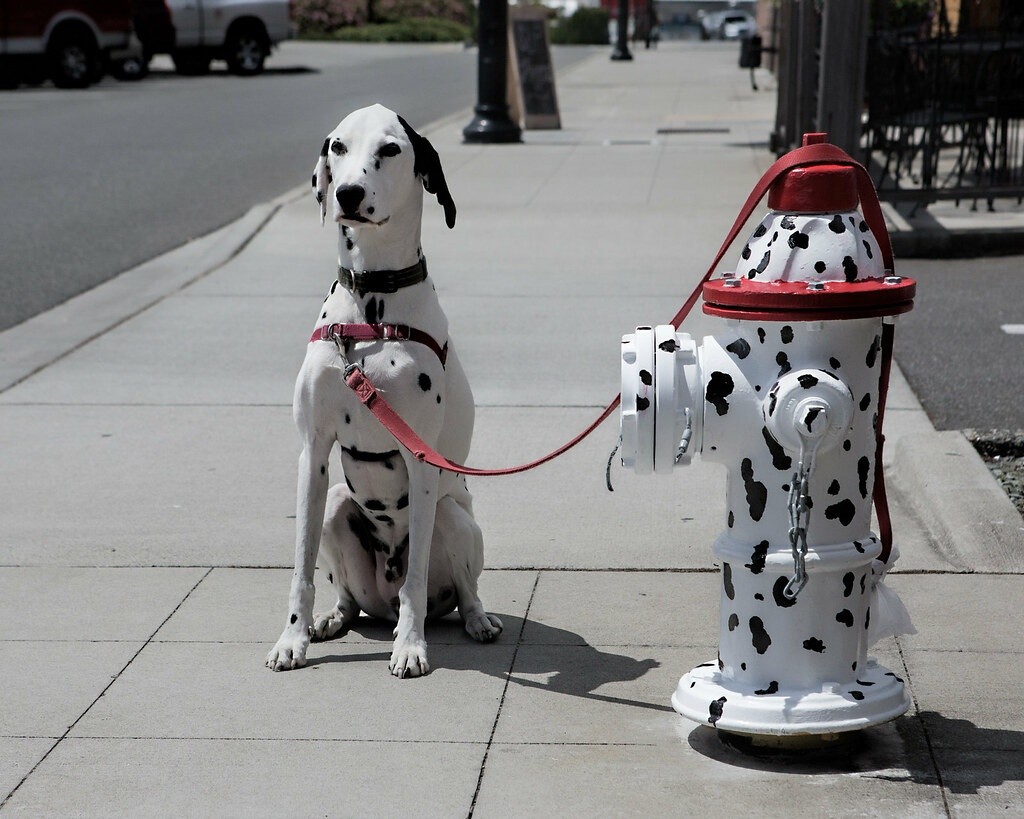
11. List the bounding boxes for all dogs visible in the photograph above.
[268,102,503,678]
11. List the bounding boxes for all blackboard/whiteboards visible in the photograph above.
[507,7,561,130]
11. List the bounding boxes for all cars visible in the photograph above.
[0,0,291,91]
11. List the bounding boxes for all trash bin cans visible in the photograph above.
[739,36,762,66]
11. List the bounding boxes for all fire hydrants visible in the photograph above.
[618,131,914,758]
